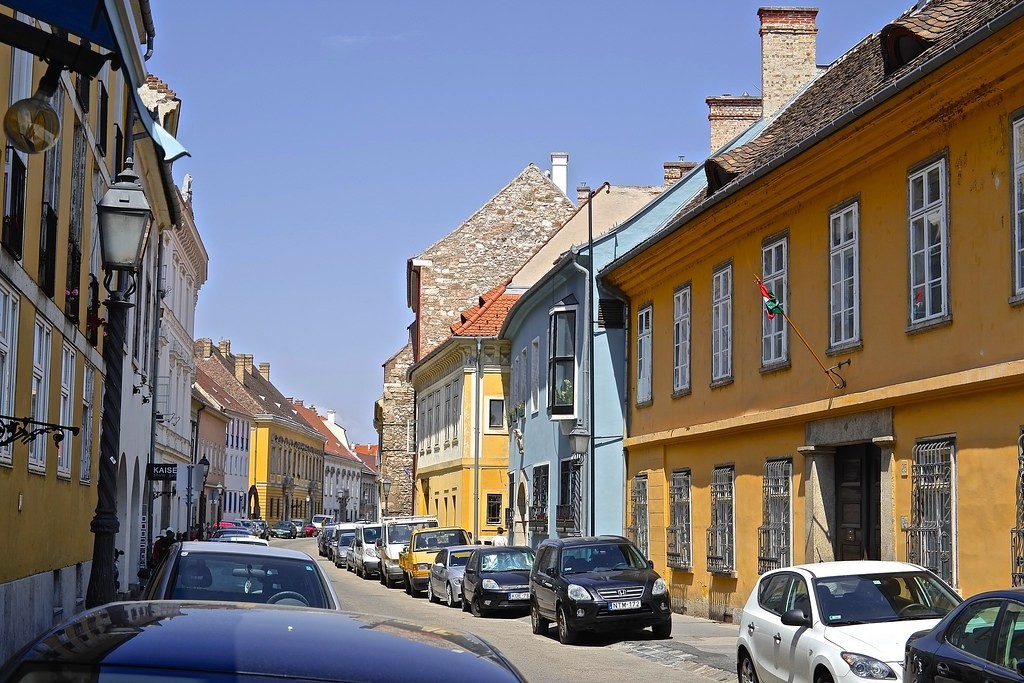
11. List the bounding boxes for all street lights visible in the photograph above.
[306,494,311,518]
[199,453,210,542]
[85,157,156,610]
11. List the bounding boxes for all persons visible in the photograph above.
[331,517,335,523]
[322,517,327,532]
[260,527,270,541]
[491,528,511,563]
[152,522,220,565]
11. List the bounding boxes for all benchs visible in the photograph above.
[965,627,1024,663]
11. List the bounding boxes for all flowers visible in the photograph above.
[66,289,79,304]
[88,308,108,337]
[556,380,573,405]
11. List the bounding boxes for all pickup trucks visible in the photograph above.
[346,514,472,598]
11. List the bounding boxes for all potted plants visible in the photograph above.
[508,407,518,423]
[504,415,512,427]
[514,400,525,417]
[161,286,173,298]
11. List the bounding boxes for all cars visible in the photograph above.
[137,542,343,613]
[902,589,1024,683]
[317,519,377,568]
[0,600,530,683]
[428,545,492,607]
[210,514,336,548]
[736,561,988,683]
[461,546,536,617]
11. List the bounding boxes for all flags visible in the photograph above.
[755,277,784,321]
[403,467,410,475]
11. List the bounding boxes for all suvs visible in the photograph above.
[528,535,672,645]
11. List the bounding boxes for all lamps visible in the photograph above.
[163,413,181,426]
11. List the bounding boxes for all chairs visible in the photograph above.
[428,538,437,546]
[175,565,213,600]
[449,536,457,544]
[817,585,845,622]
[564,555,584,572]
[261,573,291,594]
[877,578,909,616]
